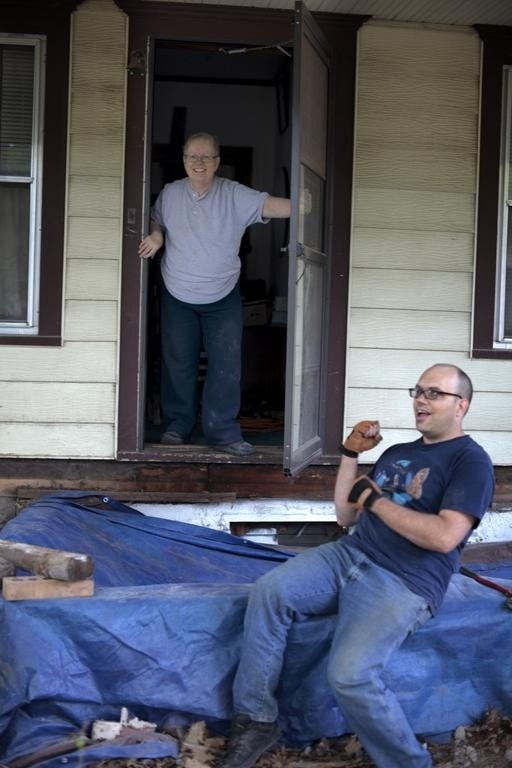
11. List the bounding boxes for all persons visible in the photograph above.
[137,132,313,456]
[220,363,494,768]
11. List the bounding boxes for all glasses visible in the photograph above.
[409,388,463,400]
[183,153,220,164]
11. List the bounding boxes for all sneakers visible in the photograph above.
[210,441,255,456]
[160,432,184,445]
[222,715,282,768]
